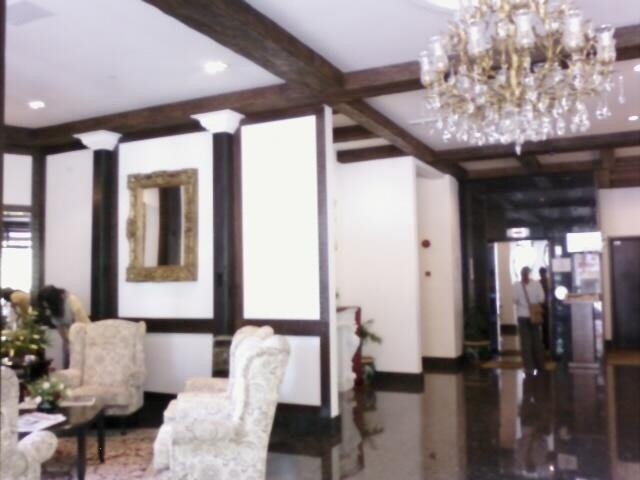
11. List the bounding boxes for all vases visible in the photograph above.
[10,348,45,370]
[37,399,57,412]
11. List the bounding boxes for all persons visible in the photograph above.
[38,283,93,370]
[538,268,551,352]
[518,364,550,475]
[511,266,547,371]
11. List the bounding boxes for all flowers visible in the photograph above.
[0,289,53,350]
[23,374,65,401]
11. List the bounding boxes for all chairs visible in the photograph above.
[145,323,290,480]
[0,366,59,480]
[50,318,146,436]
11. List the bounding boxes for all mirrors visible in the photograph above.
[124,168,198,283]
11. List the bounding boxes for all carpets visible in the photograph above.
[45,427,158,480]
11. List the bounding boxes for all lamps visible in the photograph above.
[416,0,619,156]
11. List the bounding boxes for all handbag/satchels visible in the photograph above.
[528,302,546,325]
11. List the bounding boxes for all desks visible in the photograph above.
[10,358,54,402]
